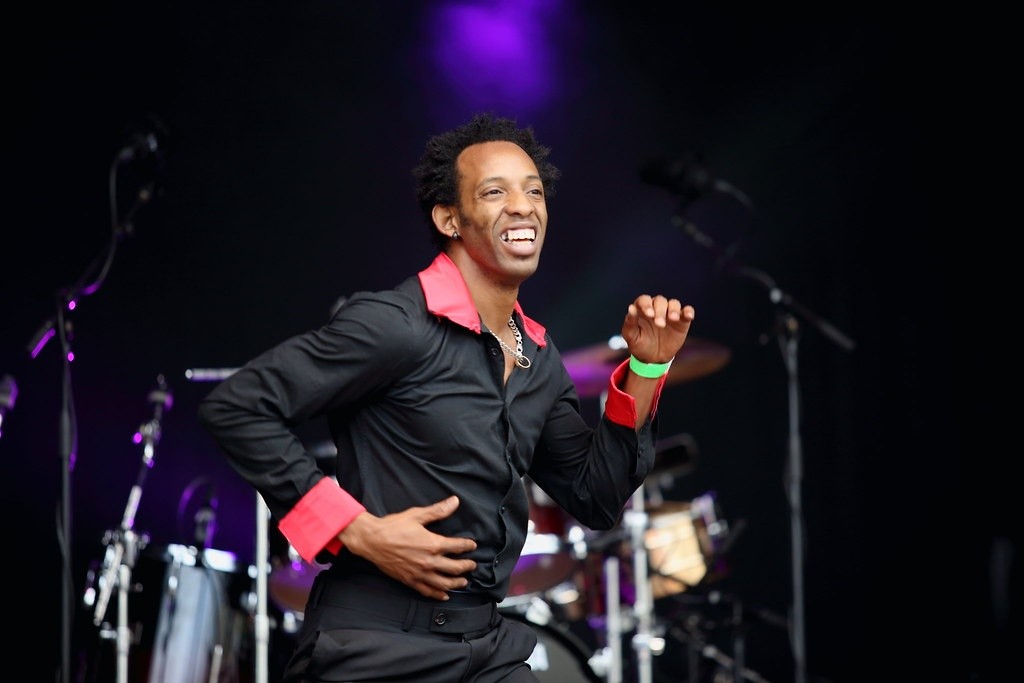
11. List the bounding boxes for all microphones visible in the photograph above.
[192,478,221,549]
[638,151,736,201]
[119,132,165,158]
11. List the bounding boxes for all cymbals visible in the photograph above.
[561,340,734,400]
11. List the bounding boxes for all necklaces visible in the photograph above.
[489,316,531,368]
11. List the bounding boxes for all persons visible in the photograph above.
[200,111,694,683]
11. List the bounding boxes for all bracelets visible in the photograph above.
[629,353,675,379]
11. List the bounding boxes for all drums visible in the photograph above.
[504,607,610,683]
[496,472,594,612]
[77,538,270,681]
